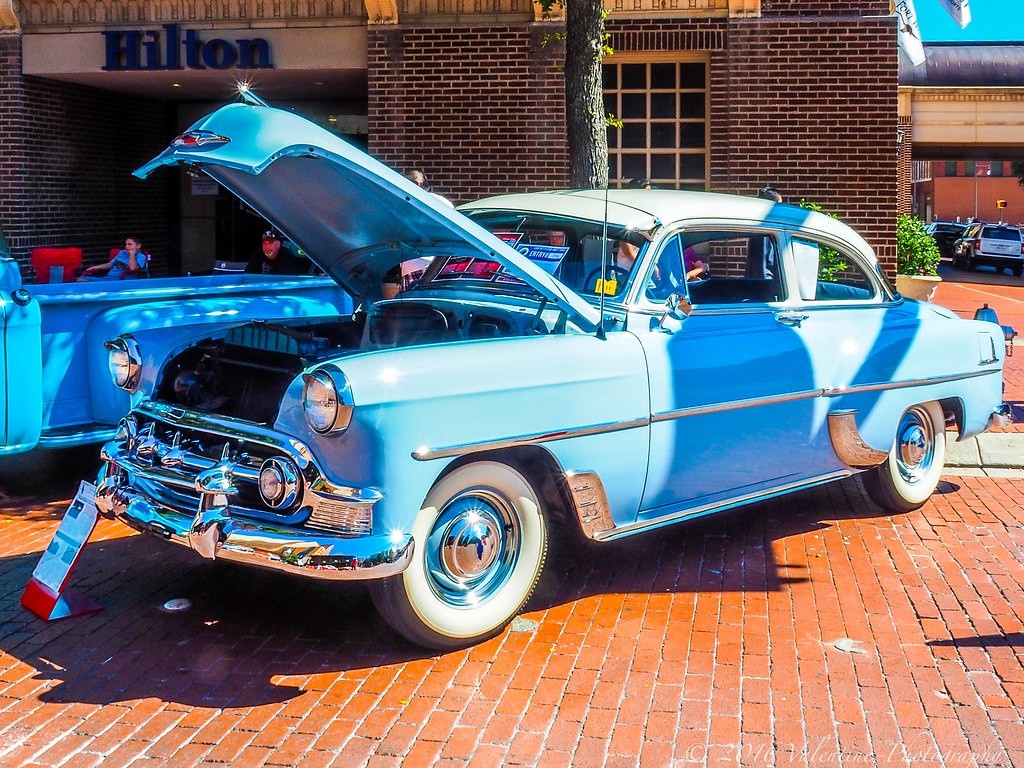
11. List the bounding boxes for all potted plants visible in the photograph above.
[897,213,942,302]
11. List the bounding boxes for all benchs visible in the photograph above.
[687,279,867,304]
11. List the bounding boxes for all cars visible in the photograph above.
[20,80,1018,655]
[918,219,1024,278]
[1,255,356,461]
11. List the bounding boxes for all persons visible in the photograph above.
[245,230,301,274]
[547,231,564,246]
[616,176,706,289]
[408,170,428,190]
[77,234,146,282]
[746,186,782,279]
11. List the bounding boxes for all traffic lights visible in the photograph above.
[996,199,1007,210]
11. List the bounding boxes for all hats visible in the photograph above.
[262,231,283,242]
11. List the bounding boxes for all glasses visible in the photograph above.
[549,235,564,239]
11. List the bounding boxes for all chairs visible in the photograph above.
[213,260,250,274]
[30,248,84,283]
[82,248,150,280]
